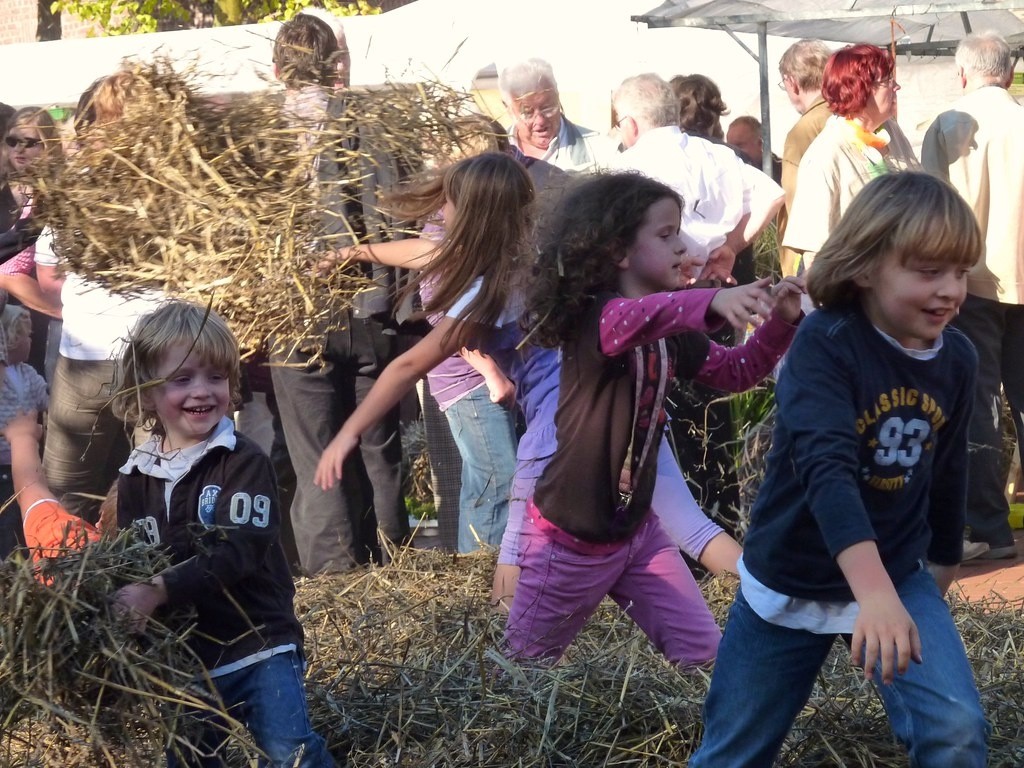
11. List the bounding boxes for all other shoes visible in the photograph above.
[975,536,1018,558]
[962,539,990,561]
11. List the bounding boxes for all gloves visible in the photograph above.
[23,498,102,586]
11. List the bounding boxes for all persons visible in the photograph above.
[1,5,1024,578]
[0,303,339,768]
[308,151,987,768]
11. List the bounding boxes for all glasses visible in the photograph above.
[778,76,790,91]
[512,103,564,119]
[615,116,627,132]
[5,135,43,148]
[872,72,894,85]
